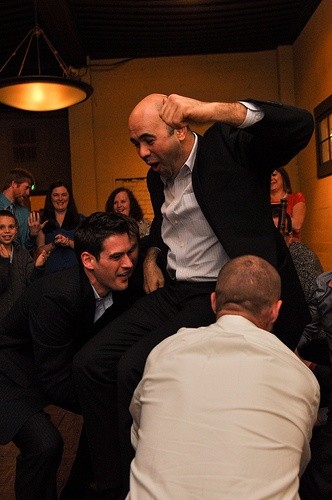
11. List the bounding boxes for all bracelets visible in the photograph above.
[49,241,56,249]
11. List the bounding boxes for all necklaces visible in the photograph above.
[282,192,287,199]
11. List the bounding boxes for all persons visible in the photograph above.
[35,182,87,282]
[125,254,322,500]
[0,168,49,257]
[269,166,305,246]
[71,94,314,500]
[0,210,50,354]
[268,205,324,361]
[317,271,332,368]
[0,211,139,500]
[104,188,150,239]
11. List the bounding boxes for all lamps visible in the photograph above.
[0,0,94,113]
